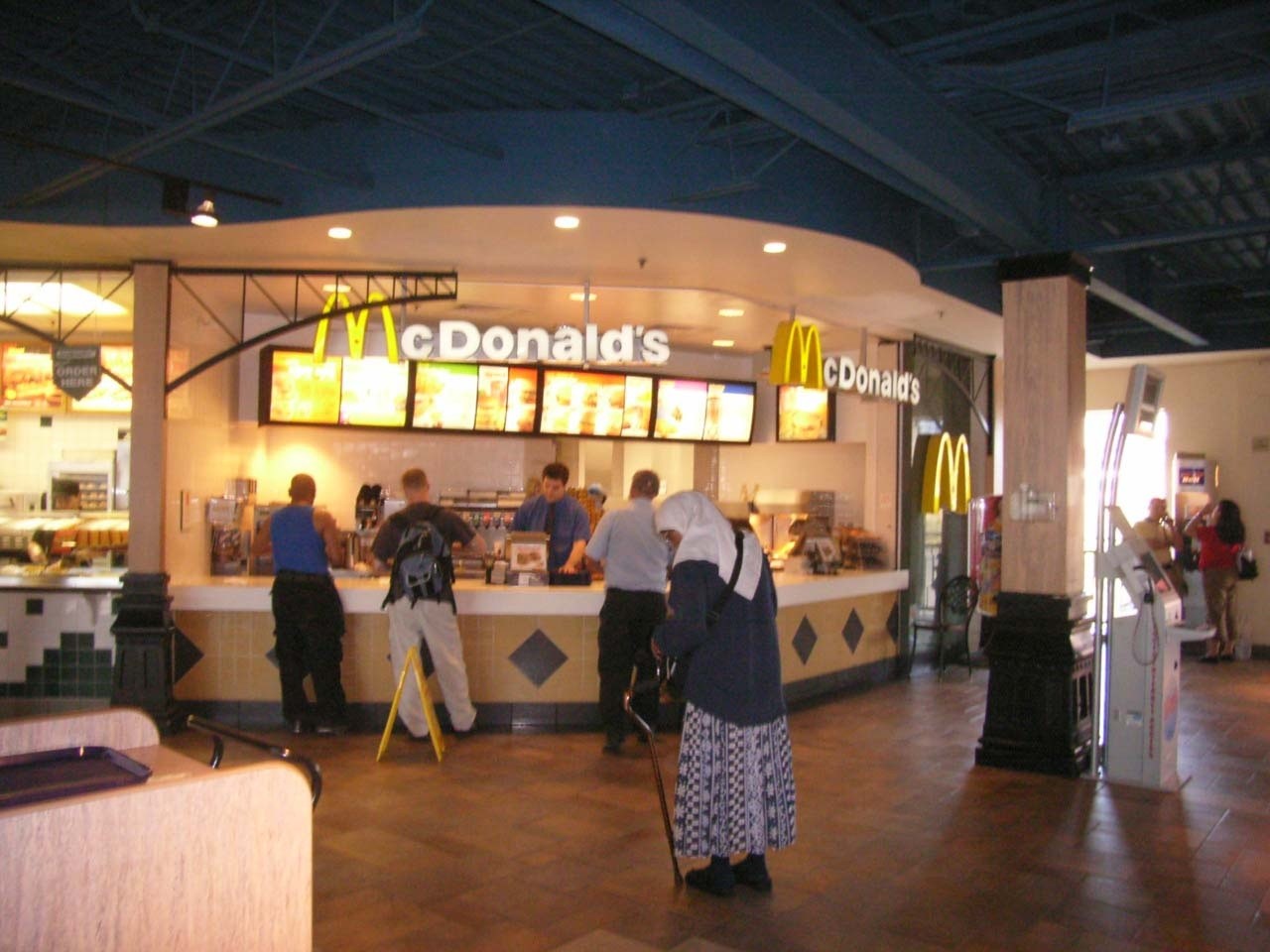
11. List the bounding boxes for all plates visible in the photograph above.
[0,746,153,807]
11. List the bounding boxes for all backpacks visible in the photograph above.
[390,504,458,610]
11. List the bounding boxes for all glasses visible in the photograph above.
[664,529,673,550]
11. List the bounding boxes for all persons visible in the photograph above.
[511,462,593,574]
[368,469,488,744]
[650,491,796,898]
[1134,497,1246,666]
[249,475,352,736]
[583,470,675,757]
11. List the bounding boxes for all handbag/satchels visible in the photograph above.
[654,649,694,688]
[1236,545,1257,581]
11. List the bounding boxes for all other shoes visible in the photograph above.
[316,718,351,734]
[683,865,734,894]
[733,862,770,893]
[602,721,633,750]
[1219,652,1234,661]
[1196,654,1221,664]
[456,721,489,736]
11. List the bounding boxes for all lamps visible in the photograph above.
[188,190,219,229]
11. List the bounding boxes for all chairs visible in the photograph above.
[907,574,980,681]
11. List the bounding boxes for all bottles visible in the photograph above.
[477,517,487,538]
[497,517,507,554]
[507,517,515,533]
[487,517,497,551]
[466,517,475,531]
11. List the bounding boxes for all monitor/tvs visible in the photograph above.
[1139,551,1170,595]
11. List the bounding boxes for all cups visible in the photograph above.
[94,558,110,578]
[491,560,506,585]
[784,556,805,578]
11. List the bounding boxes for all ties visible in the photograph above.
[543,503,557,536]
